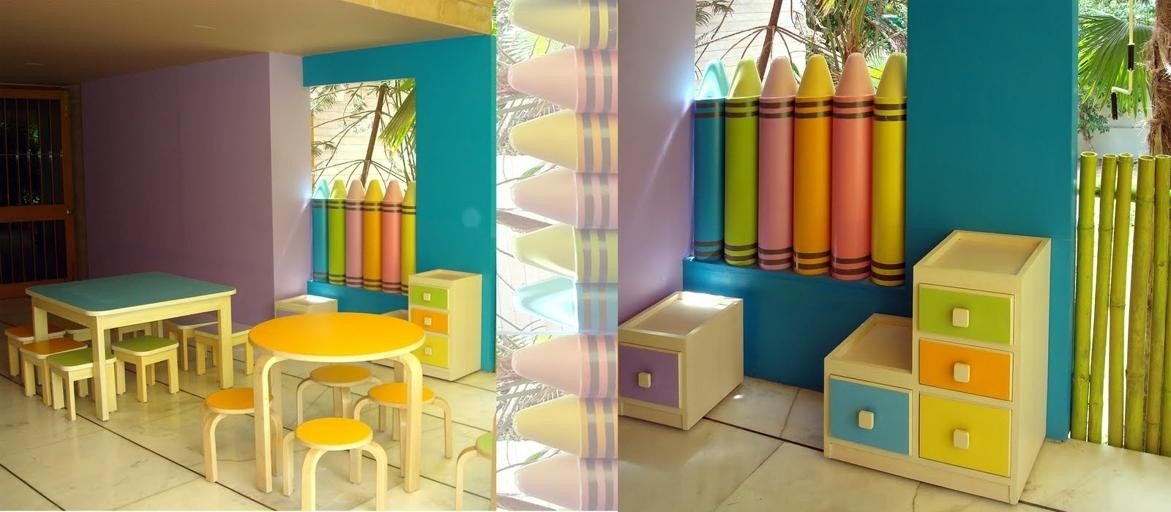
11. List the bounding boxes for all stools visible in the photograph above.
[114,323,152,341]
[4,321,66,376]
[19,337,88,407]
[112,336,179,402]
[456,433,496,510]
[46,348,117,421]
[282,417,388,511]
[296,365,385,432]
[353,382,452,478]
[165,312,218,371]
[51,316,111,353]
[194,322,254,375]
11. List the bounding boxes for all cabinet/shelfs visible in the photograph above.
[381,269,482,381]
[275,294,337,317]
[824,230,1051,505]
[201,388,283,482]
[619,291,744,431]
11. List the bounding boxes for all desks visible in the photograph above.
[248,312,426,493]
[25,272,237,421]
